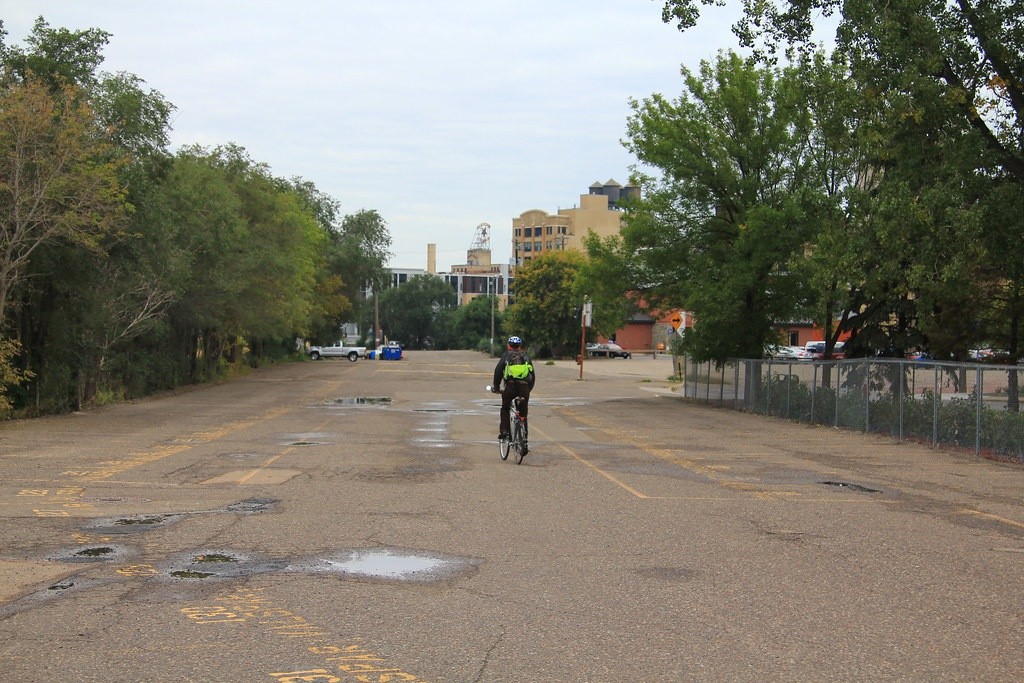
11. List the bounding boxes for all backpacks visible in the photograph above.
[503,350,534,381]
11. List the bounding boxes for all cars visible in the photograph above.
[766,341,1002,368]
[587,343,630,360]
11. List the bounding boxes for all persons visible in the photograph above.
[493,336,536,457]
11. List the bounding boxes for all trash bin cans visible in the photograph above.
[658,343,664,350]
[382,348,401,360]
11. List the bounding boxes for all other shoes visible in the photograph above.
[520,443,528,456]
[498,431,507,440]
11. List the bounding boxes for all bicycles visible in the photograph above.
[491,383,527,466]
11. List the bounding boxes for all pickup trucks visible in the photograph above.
[305,341,367,362]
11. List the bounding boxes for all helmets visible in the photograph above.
[507,336,522,346]
